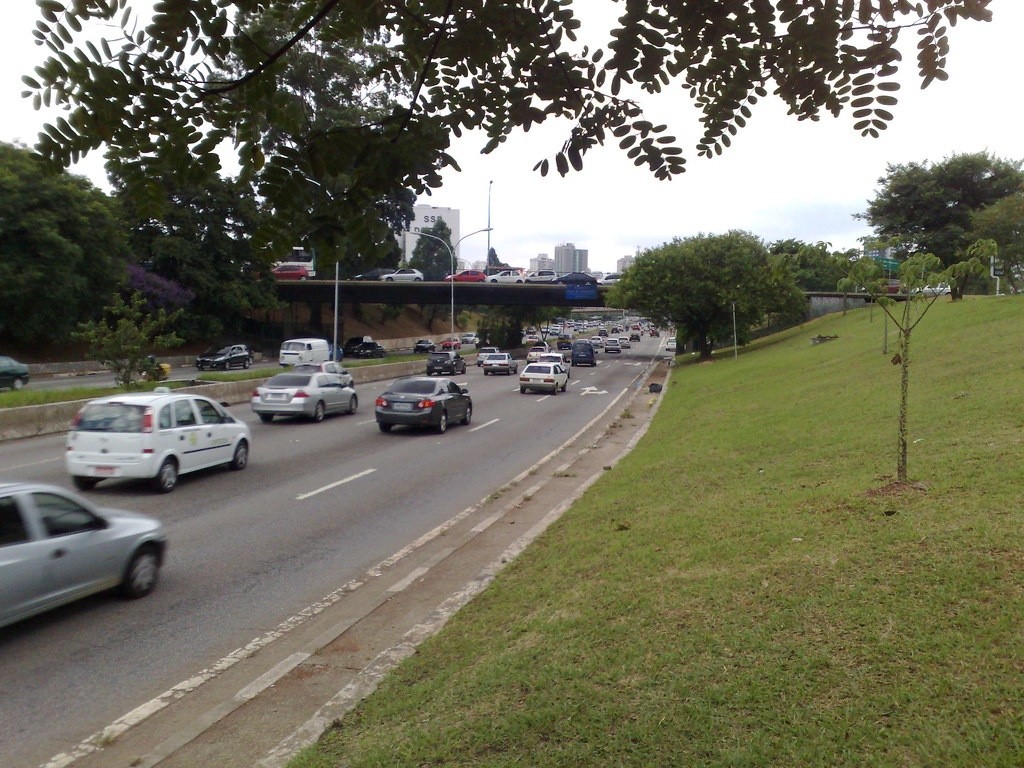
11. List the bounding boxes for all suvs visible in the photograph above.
[378,268,425,283]
[426,352,467,377]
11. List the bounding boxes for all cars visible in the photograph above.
[910,281,961,297]
[351,268,397,281]
[550,270,597,286]
[861,278,908,294]
[518,362,568,396]
[535,352,572,379]
[329,343,344,362]
[414,316,679,367]
[343,335,386,359]
[374,376,474,434]
[596,273,624,286]
[270,264,309,280]
[0,480,170,632]
[443,269,486,282]
[0,356,31,392]
[249,360,359,424]
[62,384,251,495]
[482,352,519,376]
[195,342,253,371]
[484,269,525,284]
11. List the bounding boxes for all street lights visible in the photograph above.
[411,227,494,351]
[487,179,494,277]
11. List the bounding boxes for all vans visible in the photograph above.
[524,269,557,284]
[277,337,330,370]
